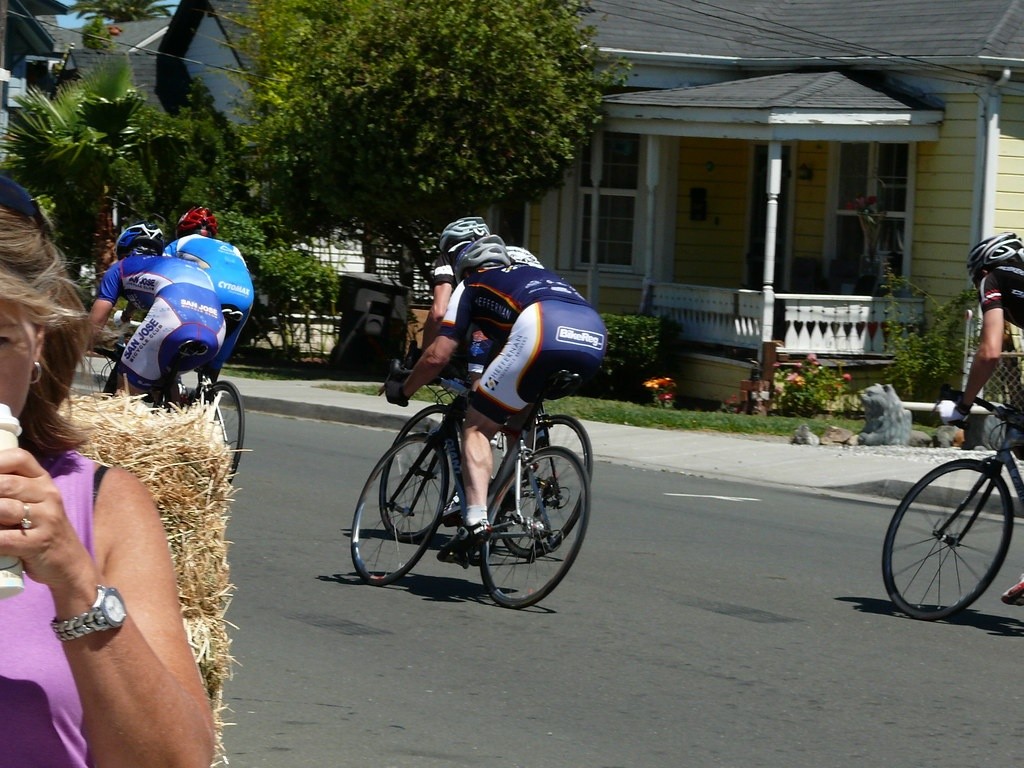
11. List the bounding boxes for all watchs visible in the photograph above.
[50,585,127,641]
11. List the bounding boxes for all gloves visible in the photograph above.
[936,398,969,427]
[385,382,410,406]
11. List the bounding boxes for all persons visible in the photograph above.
[0,175,220,768]
[85,220,227,403]
[159,206,255,383]
[385,214,606,562]
[945,229,1024,606]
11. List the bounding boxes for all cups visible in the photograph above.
[0,403,24,600]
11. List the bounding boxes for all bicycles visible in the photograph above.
[351,338,594,611]
[88,308,247,484]
[881,382,1024,622]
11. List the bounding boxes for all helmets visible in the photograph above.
[177,206,217,236]
[454,234,511,284]
[440,216,490,252]
[116,221,165,258]
[967,232,1024,282]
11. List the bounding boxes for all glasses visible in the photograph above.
[0,175,49,236]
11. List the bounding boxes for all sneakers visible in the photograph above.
[437,516,493,562]
[501,488,516,511]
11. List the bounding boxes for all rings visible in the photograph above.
[21,502,32,528]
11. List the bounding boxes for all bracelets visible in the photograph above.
[955,401,973,417]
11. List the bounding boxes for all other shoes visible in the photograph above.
[1001,574,1024,606]
[535,427,546,446]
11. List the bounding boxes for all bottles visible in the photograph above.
[489,433,498,450]
